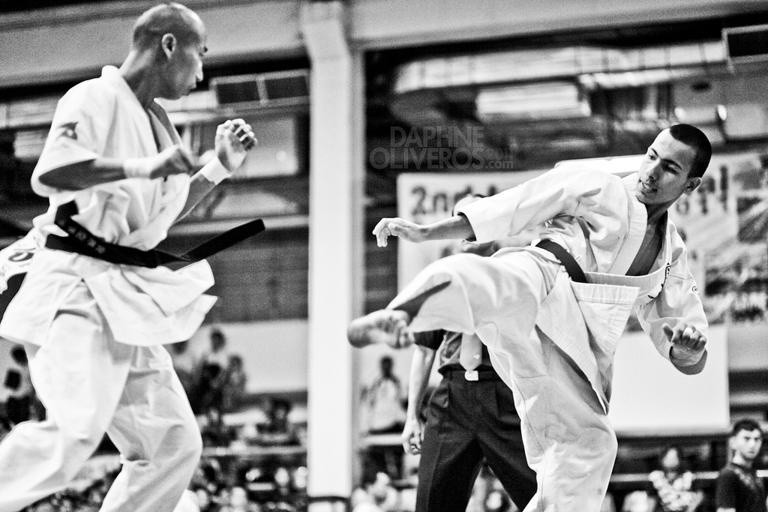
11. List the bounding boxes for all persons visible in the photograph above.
[0,3,264,512]
[402,194,537,512]
[372,125,708,512]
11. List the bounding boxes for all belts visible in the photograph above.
[444,369,499,382]
